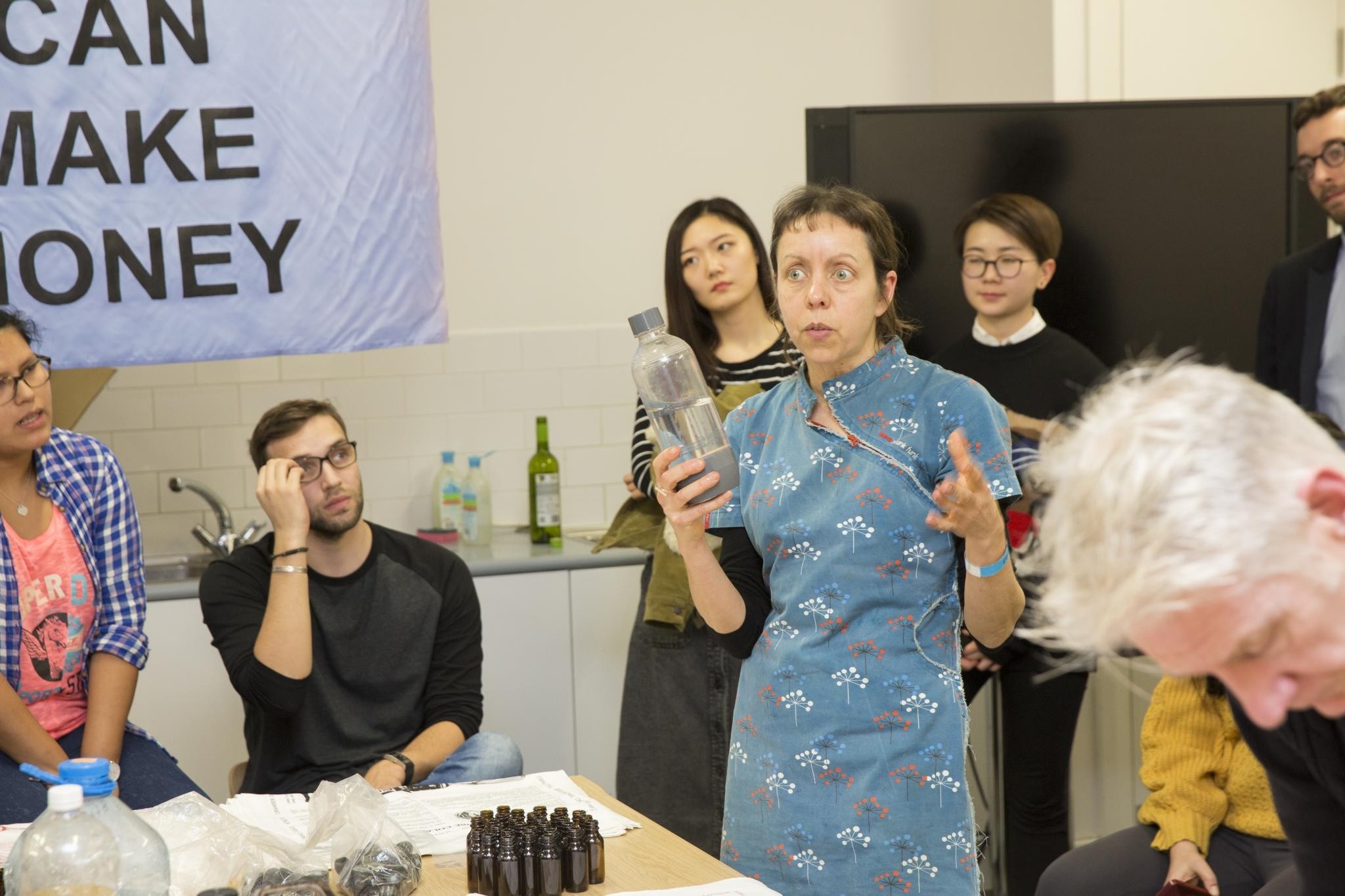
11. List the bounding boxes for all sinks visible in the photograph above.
[140,553,217,601]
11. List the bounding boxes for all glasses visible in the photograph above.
[962,253,1039,278]
[0,353,52,407]
[294,440,358,483]
[1289,142,1345,181]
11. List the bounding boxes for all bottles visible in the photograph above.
[527,415,563,542]
[459,456,493,545]
[433,451,462,532]
[19,756,171,896]
[627,306,740,505]
[3,783,119,896]
[466,806,606,895]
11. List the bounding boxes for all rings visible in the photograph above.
[654,484,676,497]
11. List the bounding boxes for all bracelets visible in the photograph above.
[270,547,310,575]
[964,550,1011,579]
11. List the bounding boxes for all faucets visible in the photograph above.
[167,476,270,558]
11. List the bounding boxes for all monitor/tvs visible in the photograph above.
[805,98,1328,379]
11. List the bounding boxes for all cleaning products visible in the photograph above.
[461,456,492,545]
[432,450,463,530]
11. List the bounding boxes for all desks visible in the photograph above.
[167,774,747,896]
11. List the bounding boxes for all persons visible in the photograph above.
[199,398,524,794]
[1019,352,1345,896]
[616,197,805,866]
[652,171,1025,896]
[1031,666,1312,896]
[0,304,215,825]
[919,193,1116,896]
[1257,78,1344,445]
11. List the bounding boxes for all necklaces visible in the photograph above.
[0,462,33,516]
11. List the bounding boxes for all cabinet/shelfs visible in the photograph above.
[126,565,648,800]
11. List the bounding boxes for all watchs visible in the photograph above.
[383,750,416,788]
